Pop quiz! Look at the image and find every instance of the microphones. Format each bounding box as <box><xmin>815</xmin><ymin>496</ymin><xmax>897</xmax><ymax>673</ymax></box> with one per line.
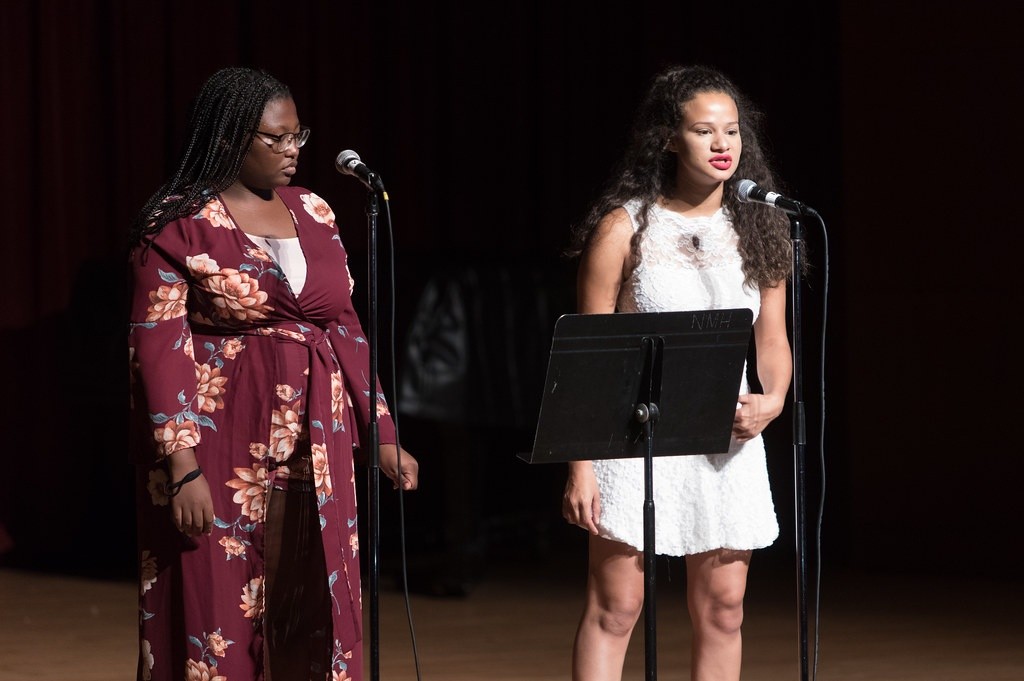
<box><xmin>733</xmin><ymin>178</ymin><xmax>818</xmax><ymax>217</ymax></box>
<box><xmin>335</xmin><ymin>150</ymin><xmax>385</xmax><ymax>193</ymax></box>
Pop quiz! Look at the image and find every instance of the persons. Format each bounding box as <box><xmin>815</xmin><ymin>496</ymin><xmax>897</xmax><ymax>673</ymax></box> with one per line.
<box><xmin>122</xmin><ymin>67</ymin><xmax>419</xmax><ymax>681</ymax></box>
<box><xmin>564</xmin><ymin>66</ymin><xmax>794</xmax><ymax>680</ymax></box>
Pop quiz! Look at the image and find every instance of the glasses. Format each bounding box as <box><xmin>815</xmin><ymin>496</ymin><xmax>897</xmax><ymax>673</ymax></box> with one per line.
<box><xmin>253</xmin><ymin>127</ymin><xmax>310</xmax><ymax>153</ymax></box>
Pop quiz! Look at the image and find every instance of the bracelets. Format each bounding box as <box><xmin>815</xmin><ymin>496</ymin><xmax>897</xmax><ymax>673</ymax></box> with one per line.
<box><xmin>163</xmin><ymin>467</ymin><xmax>201</xmax><ymax>495</ymax></box>
